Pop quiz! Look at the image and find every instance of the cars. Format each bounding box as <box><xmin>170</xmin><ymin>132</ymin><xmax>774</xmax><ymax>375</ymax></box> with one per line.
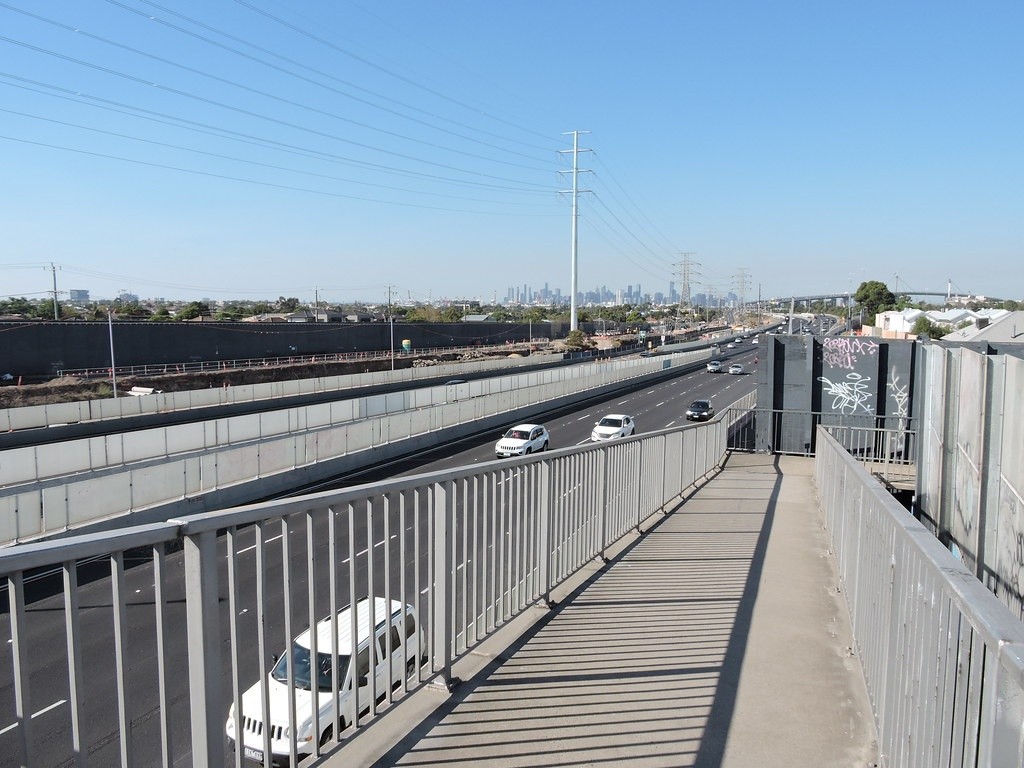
<box><xmin>494</xmin><ymin>424</ymin><xmax>550</xmax><ymax>463</ymax></box>
<box><xmin>686</xmin><ymin>400</ymin><xmax>714</xmax><ymax>422</ymax></box>
<box><xmin>754</xmin><ymin>353</ymin><xmax>759</xmax><ymax>364</ymax></box>
<box><xmin>734</xmin><ymin>338</ymin><xmax>743</xmax><ymax>343</ymax></box>
<box><xmin>744</xmin><ymin>315</ymin><xmax>789</xmax><ymax>345</ymax></box>
<box><xmin>727</xmin><ymin>343</ymin><xmax>737</xmax><ymax>349</ymax></box>
<box><xmin>802</xmin><ymin>316</ymin><xmax>835</xmax><ymax>335</ymax></box>
<box><xmin>729</xmin><ymin>364</ymin><xmax>745</xmax><ymax>375</ymax></box>
<box><xmin>591</xmin><ymin>413</ymin><xmax>635</xmax><ymax>443</ymax></box>
<box><xmin>706</xmin><ymin>361</ymin><xmax>722</xmax><ymax>373</ymax></box>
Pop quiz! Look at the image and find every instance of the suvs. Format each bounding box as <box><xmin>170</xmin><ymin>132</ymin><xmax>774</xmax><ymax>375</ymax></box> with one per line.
<box><xmin>224</xmin><ymin>595</ymin><xmax>426</xmax><ymax>768</ymax></box>
